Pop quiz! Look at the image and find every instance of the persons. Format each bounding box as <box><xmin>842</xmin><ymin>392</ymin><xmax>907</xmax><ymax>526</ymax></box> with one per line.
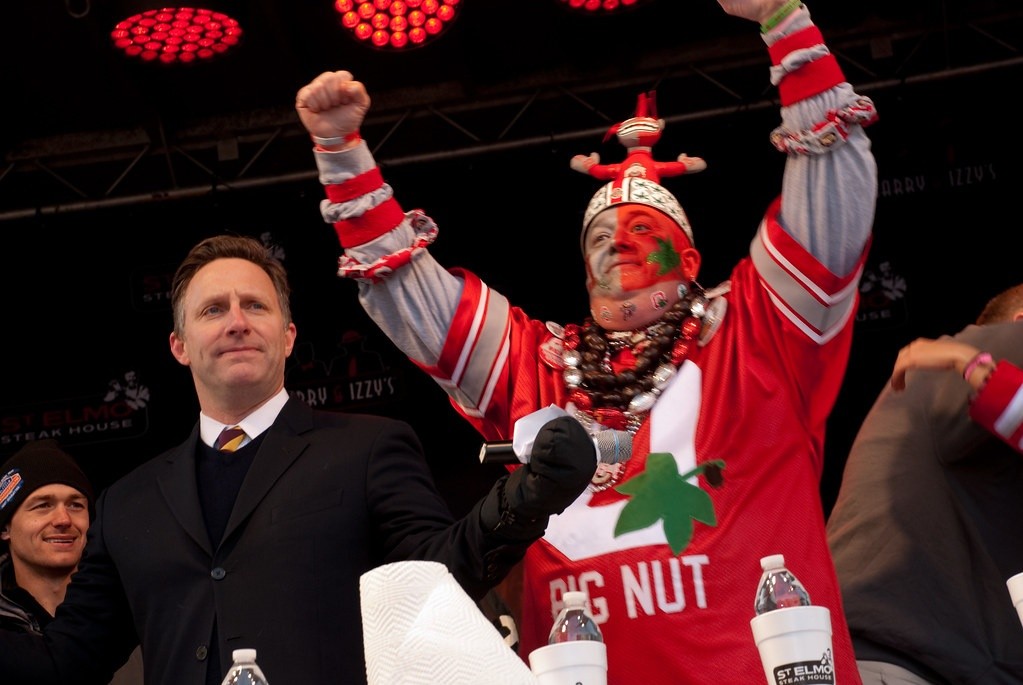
<box><xmin>824</xmin><ymin>284</ymin><xmax>1023</xmax><ymax>683</ymax></box>
<box><xmin>0</xmin><ymin>445</ymin><xmax>89</xmax><ymax>665</ymax></box>
<box><xmin>890</xmin><ymin>336</ymin><xmax>1022</xmax><ymax>452</ymax></box>
<box><xmin>0</xmin><ymin>235</ymin><xmax>595</xmax><ymax>685</ymax></box>
<box><xmin>292</xmin><ymin>0</ymin><xmax>872</xmax><ymax>685</ymax></box>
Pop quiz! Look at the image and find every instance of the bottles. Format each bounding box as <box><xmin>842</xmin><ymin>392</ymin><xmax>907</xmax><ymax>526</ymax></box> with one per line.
<box><xmin>754</xmin><ymin>554</ymin><xmax>811</xmax><ymax>616</ymax></box>
<box><xmin>222</xmin><ymin>648</ymin><xmax>269</xmax><ymax>685</ymax></box>
<box><xmin>549</xmin><ymin>592</ymin><xmax>603</xmax><ymax>643</ymax></box>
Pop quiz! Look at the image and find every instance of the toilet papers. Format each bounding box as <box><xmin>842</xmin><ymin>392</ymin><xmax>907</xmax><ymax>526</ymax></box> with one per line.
<box><xmin>360</xmin><ymin>560</ymin><xmax>539</xmax><ymax>685</ymax></box>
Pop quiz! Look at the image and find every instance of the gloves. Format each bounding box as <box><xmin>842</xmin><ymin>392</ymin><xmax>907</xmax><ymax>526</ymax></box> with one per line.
<box><xmin>481</xmin><ymin>415</ymin><xmax>597</xmax><ymax>550</ymax></box>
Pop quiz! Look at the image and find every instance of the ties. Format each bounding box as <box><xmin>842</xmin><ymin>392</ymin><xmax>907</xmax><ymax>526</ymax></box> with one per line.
<box><xmin>217</xmin><ymin>425</ymin><xmax>246</xmax><ymax>453</ymax></box>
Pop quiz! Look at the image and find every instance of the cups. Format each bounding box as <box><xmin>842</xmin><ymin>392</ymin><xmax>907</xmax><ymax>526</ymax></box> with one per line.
<box><xmin>529</xmin><ymin>640</ymin><xmax>608</xmax><ymax>685</ymax></box>
<box><xmin>750</xmin><ymin>606</ymin><xmax>836</xmax><ymax>685</ymax></box>
<box><xmin>1006</xmin><ymin>572</ymin><xmax>1023</xmax><ymax>628</ymax></box>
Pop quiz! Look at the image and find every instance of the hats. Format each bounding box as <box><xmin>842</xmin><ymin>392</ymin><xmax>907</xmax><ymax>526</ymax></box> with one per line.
<box><xmin>570</xmin><ymin>90</ymin><xmax>708</xmax><ymax>254</ymax></box>
<box><xmin>0</xmin><ymin>438</ymin><xmax>94</xmax><ymax>527</ymax></box>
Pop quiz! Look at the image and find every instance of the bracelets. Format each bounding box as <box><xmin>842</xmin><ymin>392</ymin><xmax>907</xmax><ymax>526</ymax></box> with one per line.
<box><xmin>962</xmin><ymin>350</ymin><xmax>993</xmax><ymax>383</ymax></box>
<box><xmin>760</xmin><ymin>0</ymin><xmax>805</xmax><ymax>33</ymax></box>
<box><xmin>310</xmin><ymin>130</ymin><xmax>360</xmax><ymax>146</ymax></box>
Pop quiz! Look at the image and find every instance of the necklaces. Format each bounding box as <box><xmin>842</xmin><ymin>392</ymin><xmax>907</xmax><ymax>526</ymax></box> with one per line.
<box><xmin>559</xmin><ymin>291</ymin><xmax>707</xmax><ymax>492</ymax></box>
